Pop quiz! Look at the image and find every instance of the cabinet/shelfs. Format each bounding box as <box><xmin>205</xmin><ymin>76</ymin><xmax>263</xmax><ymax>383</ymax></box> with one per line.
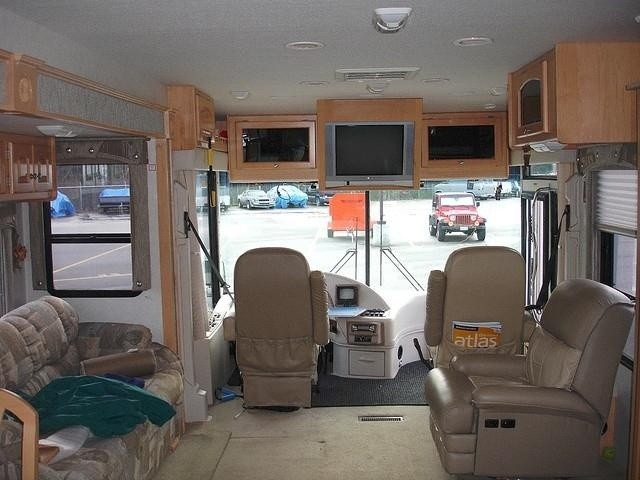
<box><xmin>508</xmin><ymin>41</ymin><xmax>640</xmax><ymax>150</ymax></box>
<box><xmin>168</xmin><ymin>86</ymin><xmax>216</xmax><ymax>151</ymax></box>
<box><xmin>226</xmin><ymin>114</ymin><xmax>319</xmax><ymax>182</ymax></box>
<box><xmin>421</xmin><ymin>112</ymin><xmax>510</xmax><ymax>180</ymax></box>
<box><xmin>0</xmin><ymin>131</ymin><xmax>57</xmax><ymax>203</ymax></box>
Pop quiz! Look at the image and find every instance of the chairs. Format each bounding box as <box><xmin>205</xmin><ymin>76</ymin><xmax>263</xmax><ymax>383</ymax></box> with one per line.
<box><xmin>0</xmin><ymin>387</ymin><xmax>39</xmax><ymax>480</ymax></box>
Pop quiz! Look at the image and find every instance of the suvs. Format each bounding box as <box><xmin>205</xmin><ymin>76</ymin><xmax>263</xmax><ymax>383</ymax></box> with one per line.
<box><xmin>429</xmin><ymin>193</ymin><xmax>487</xmax><ymax>241</ymax></box>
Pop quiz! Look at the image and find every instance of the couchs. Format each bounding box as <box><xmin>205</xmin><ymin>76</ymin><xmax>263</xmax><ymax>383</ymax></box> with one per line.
<box><xmin>424</xmin><ymin>277</ymin><xmax>637</xmax><ymax>480</ymax></box>
<box><xmin>0</xmin><ymin>296</ymin><xmax>187</xmax><ymax>480</ymax></box>
<box><xmin>221</xmin><ymin>247</ymin><xmax>330</xmax><ymax>409</ymax></box>
<box><xmin>425</xmin><ymin>247</ymin><xmax>537</xmax><ymax>369</ymax></box>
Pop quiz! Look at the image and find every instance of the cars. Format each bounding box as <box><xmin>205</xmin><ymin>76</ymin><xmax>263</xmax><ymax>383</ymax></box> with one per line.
<box><xmin>268</xmin><ymin>185</ymin><xmax>309</xmax><ymax>208</ymax></box>
<box><xmin>236</xmin><ymin>190</ymin><xmax>275</xmax><ymax>209</ymax></box>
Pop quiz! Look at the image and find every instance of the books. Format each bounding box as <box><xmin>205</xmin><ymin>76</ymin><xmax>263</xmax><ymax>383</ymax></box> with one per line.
<box><xmin>327</xmin><ymin>307</ymin><xmax>366</xmax><ymax>317</ymax></box>
<box><xmin>452</xmin><ymin>321</ymin><xmax>502</xmax><ymax>348</ymax></box>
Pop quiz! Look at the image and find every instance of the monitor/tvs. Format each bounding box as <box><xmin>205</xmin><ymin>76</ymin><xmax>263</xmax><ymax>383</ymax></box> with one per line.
<box><xmin>323</xmin><ymin>119</ymin><xmax>416</xmax><ymax>188</ymax></box>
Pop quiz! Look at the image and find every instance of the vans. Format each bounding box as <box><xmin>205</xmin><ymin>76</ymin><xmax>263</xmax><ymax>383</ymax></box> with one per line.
<box><xmin>433</xmin><ymin>180</ymin><xmax>502</xmax><ymax>200</ymax></box>
<box><xmin>305</xmin><ymin>190</ymin><xmax>333</xmax><ymax>207</ymax></box>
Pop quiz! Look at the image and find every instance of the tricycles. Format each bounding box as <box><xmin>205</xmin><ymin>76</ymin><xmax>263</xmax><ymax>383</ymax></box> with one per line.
<box><xmin>326</xmin><ymin>194</ymin><xmax>376</xmax><ymax>239</ymax></box>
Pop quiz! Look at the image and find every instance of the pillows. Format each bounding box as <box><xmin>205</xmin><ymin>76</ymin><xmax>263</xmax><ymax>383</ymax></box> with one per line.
<box><xmin>81</xmin><ymin>347</ymin><xmax>156</xmax><ymax>379</ymax></box>
